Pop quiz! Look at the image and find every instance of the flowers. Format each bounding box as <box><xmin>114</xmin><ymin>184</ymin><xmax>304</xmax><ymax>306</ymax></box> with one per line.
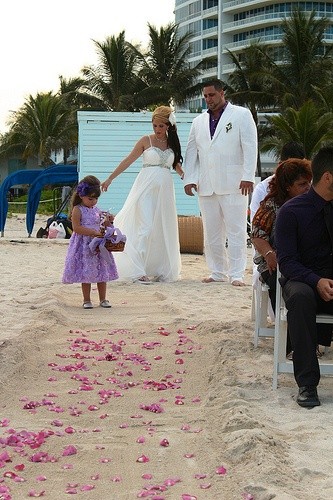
<box><xmin>169</xmin><ymin>112</ymin><xmax>177</xmax><ymax>125</ymax></box>
<box><xmin>76</xmin><ymin>182</ymin><xmax>87</xmax><ymax>194</ymax></box>
<box><xmin>224</xmin><ymin>123</ymin><xmax>233</xmax><ymax>133</ymax></box>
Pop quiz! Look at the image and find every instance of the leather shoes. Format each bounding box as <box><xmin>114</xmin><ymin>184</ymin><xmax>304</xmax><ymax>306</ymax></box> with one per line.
<box><xmin>297</xmin><ymin>386</ymin><xmax>320</xmax><ymax>406</ymax></box>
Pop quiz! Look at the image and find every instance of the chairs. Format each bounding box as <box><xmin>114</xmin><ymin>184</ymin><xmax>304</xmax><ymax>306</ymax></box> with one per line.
<box><xmin>249</xmin><ymin>173</ymin><xmax>333</xmax><ymax>389</ymax></box>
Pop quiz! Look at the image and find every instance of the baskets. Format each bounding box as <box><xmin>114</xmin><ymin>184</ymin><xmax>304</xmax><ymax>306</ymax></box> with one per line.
<box><xmin>95</xmin><ymin>225</ymin><xmax>126</xmax><ymax>251</ymax></box>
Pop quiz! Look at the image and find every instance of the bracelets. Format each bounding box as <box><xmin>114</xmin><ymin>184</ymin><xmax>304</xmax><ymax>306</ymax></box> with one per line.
<box><xmin>265</xmin><ymin>250</ymin><xmax>275</xmax><ymax>259</ymax></box>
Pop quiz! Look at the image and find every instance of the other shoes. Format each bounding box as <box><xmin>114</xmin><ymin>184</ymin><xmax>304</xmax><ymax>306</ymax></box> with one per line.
<box><xmin>100</xmin><ymin>300</ymin><xmax>111</xmax><ymax>307</ymax></box>
<box><xmin>83</xmin><ymin>302</ymin><xmax>92</xmax><ymax>309</ymax></box>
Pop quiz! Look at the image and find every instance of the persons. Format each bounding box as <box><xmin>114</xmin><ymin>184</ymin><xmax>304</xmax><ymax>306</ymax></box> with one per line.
<box><xmin>272</xmin><ymin>147</ymin><xmax>333</xmax><ymax>408</ymax></box>
<box><xmin>101</xmin><ymin>106</ymin><xmax>184</xmax><ymax>284</ymax></box>
<box><xmin>183</xmin><ymin>78</ymin><xmax>258</xmax><ymax>286</ymax></box>
<box><xmin>61</xmin><ymin>175</ymin><xmax>120</xmax><ymax>309</ymax></box>
<box><xmin>250</xmin><ymin>158</ymin><xmax>313</xmax><ymax>360</ymax></box>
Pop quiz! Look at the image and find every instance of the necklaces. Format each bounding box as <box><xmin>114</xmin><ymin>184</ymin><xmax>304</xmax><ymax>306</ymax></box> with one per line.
<box><xmin>154</xmin><ymin>134</ymin><xmax>168</xmax><ymax>142</ymax></box>
